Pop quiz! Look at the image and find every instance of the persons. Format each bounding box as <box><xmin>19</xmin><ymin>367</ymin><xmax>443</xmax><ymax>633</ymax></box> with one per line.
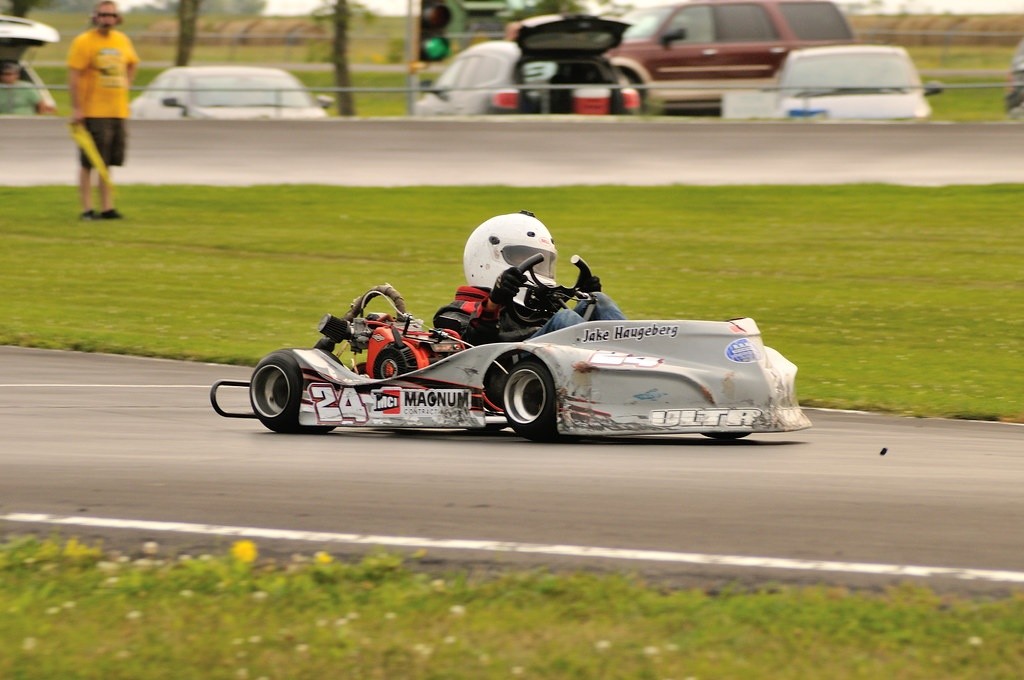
<box><xmin>1008</xmin><ymin>37</ymin><xmax>1024</xmax><ymax>120</ymax></box>
<box><xmin>0</xmin><ymin>63</ymin><xmax>41</xmax><ymax>116</ymax></box>
<box><xmin>65</xmin><ymin>0</ymin><xmax>138</xmax><ymax>220</ymax></box>
<box><xmin>432</xmin><ymin>210</ymin><xmax>627</xmax><ymax>349</ymax></box>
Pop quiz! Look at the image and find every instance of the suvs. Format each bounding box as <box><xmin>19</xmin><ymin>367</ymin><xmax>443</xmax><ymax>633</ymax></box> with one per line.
<box><xmin>416</xmin><ymin>12</ymin><xmax>643</xmax><ymax>115</ymax></box>
<box><xmin>603</xmin><ymin>1</ymin><xmax>856</xmax><ymax>104</ymax></box>
<box><xmin>0</xmin><ymin>16</ymin><xmax>60</xmax><ymax>117</ymax></box>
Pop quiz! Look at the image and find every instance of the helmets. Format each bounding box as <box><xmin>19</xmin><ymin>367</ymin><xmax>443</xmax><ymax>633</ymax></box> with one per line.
<box><xmin>464</xmin><ymin>212</ymin><xmax>557</xmax><ymax>306</ymax></box>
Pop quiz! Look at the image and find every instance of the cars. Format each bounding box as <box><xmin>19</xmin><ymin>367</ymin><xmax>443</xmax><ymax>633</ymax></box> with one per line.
<box><xmin>762</xmin><ymin>46</ymin><xmax>944</xmax><ymax>121</ymax></box>
<box><xmin>128</xmin><ymin>65</ymin><xmax>335</xmax><ymax>121</ymax></box>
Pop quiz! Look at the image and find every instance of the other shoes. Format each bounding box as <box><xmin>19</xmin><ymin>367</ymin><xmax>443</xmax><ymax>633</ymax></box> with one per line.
<box><xmin>82</xmin><ymin>210</ymin><xmax>94</xmax><ymax>219</ymax></box>
<box><xmin>99</xmin><ymin>208</ymin><xmax>122</xmax><ymax>218</ymax></box>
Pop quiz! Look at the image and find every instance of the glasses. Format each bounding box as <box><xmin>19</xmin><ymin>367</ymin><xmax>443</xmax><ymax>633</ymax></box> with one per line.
<box><xmin>98</xmin><ymin>12</ymin><xmax>118</xmax><ymax>18</ymax></box>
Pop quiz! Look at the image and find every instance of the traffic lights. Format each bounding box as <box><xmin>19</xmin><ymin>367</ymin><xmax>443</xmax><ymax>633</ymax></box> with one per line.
<box><xmin>411</xmin><ymin>1</ymin><xmax>453</xmax><ymax>64</ymax></box>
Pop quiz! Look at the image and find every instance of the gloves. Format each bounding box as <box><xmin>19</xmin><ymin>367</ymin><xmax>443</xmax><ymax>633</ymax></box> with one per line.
<box><xmin>576</xmin><ymin>276</ymin><xmax>602</xmax><ymax>294</ymax></box>
<box><xmin>489</xmin><ymin>266</ymin><xmax>528</xmax><ymax>307</ymax></box>
